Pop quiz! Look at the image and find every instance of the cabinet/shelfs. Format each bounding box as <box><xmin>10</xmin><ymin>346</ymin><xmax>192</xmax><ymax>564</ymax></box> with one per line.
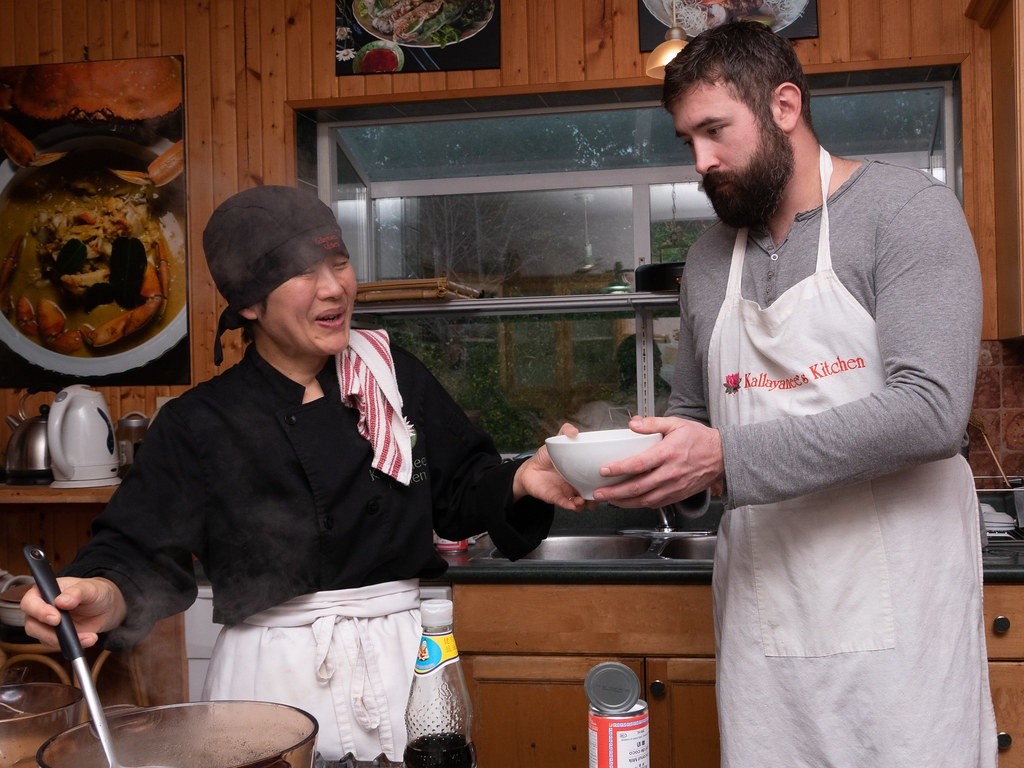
<box><xmin>452</xmin><ymin>584</ymin><xmax>1024</xmax><ymax>768</ymax></box>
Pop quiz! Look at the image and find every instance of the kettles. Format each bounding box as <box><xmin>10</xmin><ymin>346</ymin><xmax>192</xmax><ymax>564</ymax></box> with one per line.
<box><xmin>1</xmin><ymin>385</ymin><xmax>65</xmax><ymax>485</ymax></box>
<box><xmin>46</xmin><ymin>384</ymin><xmax>123</xmax><ymax>487</ymax></box>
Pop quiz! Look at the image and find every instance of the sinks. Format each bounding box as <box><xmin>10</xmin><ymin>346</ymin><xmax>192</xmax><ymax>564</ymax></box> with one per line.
<box><xmin>466</xmin><ymin>530</ymin><xmax>658</xmax><ymax>563</ymax></box>
<box><xmin>658</xmin><ymin>531</ymin><xmax>719</xmax><ymax>563</ymax></box>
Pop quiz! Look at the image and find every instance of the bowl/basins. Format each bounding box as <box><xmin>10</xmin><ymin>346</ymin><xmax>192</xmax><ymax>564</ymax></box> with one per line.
<box><xmin>35</xmin><ymin>700</ymin><xmax>319</xmax><ymax>768</ymax></box>
<box><xmin>0</xmin><ymin>681</ymin><xmax>86</xmax><ymax>768</ymax></box>
<box><xmin>545</xmin><ymin>427</ymin><xmax>666</xmax><ymax>501</ymax></box>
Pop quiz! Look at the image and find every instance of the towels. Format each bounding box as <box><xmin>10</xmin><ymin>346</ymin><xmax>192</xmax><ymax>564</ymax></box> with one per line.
<box><xmin>331</xmin><ymin>322</ymin><xmax>417</xmax><ymax>486</ymax></box>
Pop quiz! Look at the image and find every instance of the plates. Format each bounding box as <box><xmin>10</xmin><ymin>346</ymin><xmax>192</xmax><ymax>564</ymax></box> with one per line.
<box><xmin>980</xmin><ymin>503</ymin><xmax>1017</xmax><ymax>532</ymax></box>
<box><xmin>0</xmin><ymin>137</ymin><xmax>187</xmax><ymax>380</ymax></box>
<box><xmin>640</xmin><ymin>0</ymin><xmax>810</xmax><ymax>42</ymax></box>
<box><xmin>351</xmin><ymin>0</ymin><xmax>496</xmax><ymax>47</ymax></box>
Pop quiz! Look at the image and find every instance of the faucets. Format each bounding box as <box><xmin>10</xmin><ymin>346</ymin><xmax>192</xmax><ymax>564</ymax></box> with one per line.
<box><xmin>657</xmin><ymin>506</ymin><xmax>675</xmax><ymax>532</ymax></box>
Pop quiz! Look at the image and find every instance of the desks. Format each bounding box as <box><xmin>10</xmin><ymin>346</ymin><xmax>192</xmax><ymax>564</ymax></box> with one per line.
<box><xmin>0</xmin><ymin>484</ymin><xmax>189</xmax><ymax>707</ymax></box>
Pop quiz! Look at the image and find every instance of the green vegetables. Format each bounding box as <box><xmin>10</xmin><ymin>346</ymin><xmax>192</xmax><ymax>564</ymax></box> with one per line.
<box><xmin>404</xmin><ymin>0</ymin><xmax>490</xmax><ymax>48</ymax></box>
<box><xmin>395</xmin><ymin>325</ymin><xmax>548</xmax><ymax>452</ymax></box>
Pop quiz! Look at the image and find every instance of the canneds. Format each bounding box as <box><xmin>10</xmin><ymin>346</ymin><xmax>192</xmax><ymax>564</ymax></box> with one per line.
<box><xmin>582</xmin><ymin>660</ymin><xmax>650</xmax><ymax>768</ymax></box>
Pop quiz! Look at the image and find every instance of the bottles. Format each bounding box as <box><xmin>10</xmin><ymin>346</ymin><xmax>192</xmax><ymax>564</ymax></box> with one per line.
<box><xmin>403</xmin><ymin>597</ymin><xmax>479</xmax><ymax>767</ymax></box>
<box><xmin>116</xmin><ymin>418</ymin><xmax>149</xmax><ymax>477</ymax></box>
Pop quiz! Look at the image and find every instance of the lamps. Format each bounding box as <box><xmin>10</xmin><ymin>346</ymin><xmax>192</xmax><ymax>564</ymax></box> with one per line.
<box><xmin>604</xmin><ymin>261</ymin><xmax>634</xmax><ymax>294</ymax></box>
<box><xmin>646</xmin><ymin>0</ymin><xmax>689</xmax><ymax>79</ymax></box>
<box><xmin>574</xmin><ymin>190</ymin><xmax>600</xmax><ymax>273</ymax></box>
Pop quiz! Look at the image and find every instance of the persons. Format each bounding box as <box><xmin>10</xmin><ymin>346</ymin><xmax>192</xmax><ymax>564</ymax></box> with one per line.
<box><xmin>586</xmin><ymin>20</ymin><xmax>996</xmax><ymax>768</ymax></box>
<box><xmin>19</xmin><ymin>184</ymin><xmax>592</xmax><ymax>766</ymax></box>
<box><xmin>609</xmin><ymin>332</ymin><xmax>676</xmax><ymax>421</ymax></box>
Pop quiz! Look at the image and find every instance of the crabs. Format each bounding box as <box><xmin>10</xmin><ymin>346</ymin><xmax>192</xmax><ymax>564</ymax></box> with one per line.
<box><xmin>0</xmin><ymin>57</ymin><xmax>185</xmax><ymax>188</ymax></box>
<box><xmin>0</xmin><ymin>233</ymin><xmax>170</xmax><ymax>351</ymax></box>
